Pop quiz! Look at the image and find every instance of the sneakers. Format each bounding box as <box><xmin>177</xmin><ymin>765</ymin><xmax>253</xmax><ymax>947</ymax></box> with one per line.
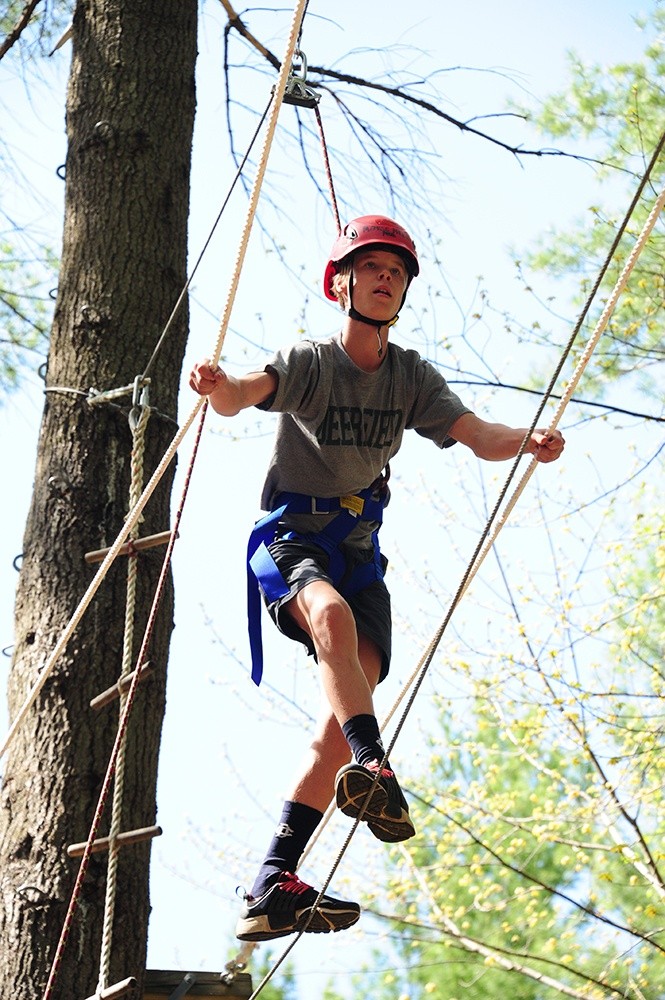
<box><xmin>236</xmin><ymin>872</ymin><xmax>361</xmax><ymax>941</ymax></box>
<box><xmin>334</xmin><ymin>763</ymin><xmax>416</xmax><ymax>842</ymax></box>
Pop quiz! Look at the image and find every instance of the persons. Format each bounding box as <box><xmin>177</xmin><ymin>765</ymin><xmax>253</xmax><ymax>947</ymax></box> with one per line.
<box><xmin>189</xmin><ymin>215</ymin><xmax>566</xmax><ymax>942</ymax></box>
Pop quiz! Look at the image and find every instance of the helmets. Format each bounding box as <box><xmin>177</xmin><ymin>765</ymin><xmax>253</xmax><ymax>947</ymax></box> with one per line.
<box><xmin>324</xmin><ymin>215</ymin><xmax>419</xmax><ymax>300</ymax></box>
